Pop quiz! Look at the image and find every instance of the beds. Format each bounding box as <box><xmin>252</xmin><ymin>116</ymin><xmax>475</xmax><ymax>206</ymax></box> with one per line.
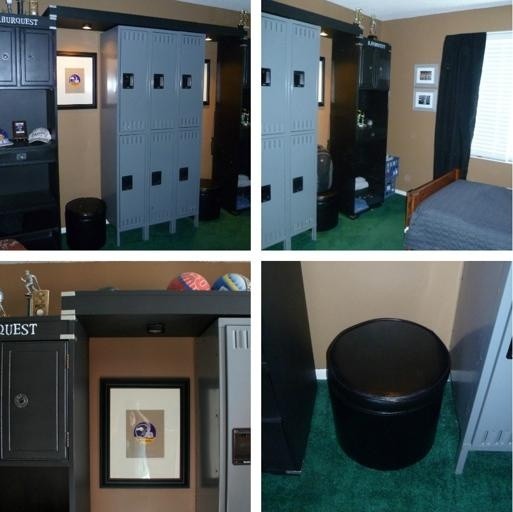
<box><xmin>399</xmin><ymin>167</ymin><xmax>512</xmax><ymax>252</ymax></box>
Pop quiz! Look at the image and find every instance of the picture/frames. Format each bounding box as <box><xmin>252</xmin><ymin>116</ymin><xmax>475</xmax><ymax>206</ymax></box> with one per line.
<box><xmin>317</xmin><ymin>56</ymin><xmax>325</xmax><ymax>108</ymax></box>
<box><xmin>54</xmin><ymin>49</ymin><xmax>98</xmax><ymax>111</ymax></box>
<box><xmin>412</xmin><ymin>62</ymin><xmax>439</xmax><ymax>89</ymax></box>
<box><xmin>96</xmin><ymin>372</ymin><xmax>191</xmax><ymax>489</ymax></box>
<box><xmin>411</xmin><ymin>87</ymin><xmax>438</xmax><ymax>114</ymax></box>
<box><xmin>200</xmin><ymin>57</ymin><xmax>211</xmax><ymax>107</ymax></box>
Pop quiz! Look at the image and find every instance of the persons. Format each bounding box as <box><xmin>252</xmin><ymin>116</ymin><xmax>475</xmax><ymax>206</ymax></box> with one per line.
<box><xmin>21</xmin><ymin>269</ymin><xmax>37</xmax><ymax>295</ymax></box>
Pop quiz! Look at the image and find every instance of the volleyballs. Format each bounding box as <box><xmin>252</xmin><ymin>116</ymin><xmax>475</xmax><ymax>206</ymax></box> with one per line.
<box><xmin>134</xmin><ymin>422</ymin><xmax>157</xmax><ymax>443</ymax></box>
<box><xmin>69</xmin><ymin>74</ymin><xmax>80</xmax><ymax>88</ymax></box>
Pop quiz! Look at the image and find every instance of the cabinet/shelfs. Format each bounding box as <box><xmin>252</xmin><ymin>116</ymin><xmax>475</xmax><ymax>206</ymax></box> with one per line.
<box><xmin>0</xmin><ymin>4</ymin><xmax>63</xmax><ymax>252</ymax></box>
<box><xmin>94</xmin><ymin>23</ymin><xmax>207</xmax><ymax>247</ymax></box>
<box><xmin>190</xmin><ymin>316</ymin><xmax>251</xmax><ymax>512</ymax></box>
<box><xmin>0</xmin><ymin>313</ymin><xmax>91</xmax><ymax>511</ymax></box>
<box><xmin>327</xmin><ymin>34</ymin><xmax>392</xmax><ymax>220</ymax></box>
<box><xmin>447</xmin><ymin>260</ymin><xmax>512</xmax><ymax>477</ymax></box>
<box><xmin>257</xmin><ymin>11</ymin><xmax>324</xmax><ymax>251</ymax></box>
<box><xmin>259</xmin><ymin>260</ymin><xmax>318</xmax><ymax>477</ymax></box>
<box><xmin>210</xmin><ymin>36</ymin><xmax>250</xmax><ymax>217</ymax></box>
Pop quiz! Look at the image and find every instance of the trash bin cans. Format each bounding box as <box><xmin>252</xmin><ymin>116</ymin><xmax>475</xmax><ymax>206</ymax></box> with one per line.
<box><xmin>308</xmin><ymin>189</ymin><xmax>339</xmax><ymax>232</ymax></box>
<box><xmin>189</xmin><ymin>178</ymin><xmax>220</xmax><ymax>221</ymax></box>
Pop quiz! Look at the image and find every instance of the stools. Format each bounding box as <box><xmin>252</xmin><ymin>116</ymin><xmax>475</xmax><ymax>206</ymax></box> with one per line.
<box><xmin>315</xmin><ymin>186</ymin><xmax>339</xmax><ymax>234</ymax></box>
<box><xmin>186</xmin><ymin>176</ymin><xmax>223</xmax><ymax>222</ymax></box>
